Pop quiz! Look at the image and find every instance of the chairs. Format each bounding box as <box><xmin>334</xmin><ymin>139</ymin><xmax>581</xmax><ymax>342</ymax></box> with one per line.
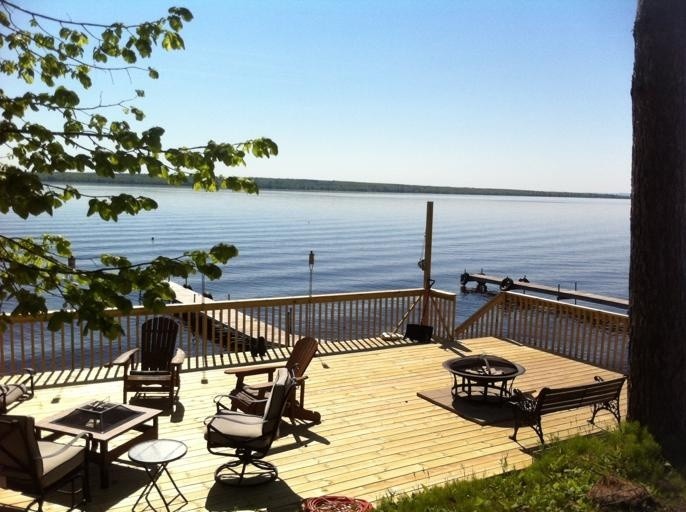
<box><xmin>0</xmin><ymin>413</ymin><xmax>97</xmax><ymax>512</ymax></box>
<box><xmin>0</xmin><ymin>366</ymin><xmax>36</xmax><ymax>415</ymax></box>
<box><xmin>112</xmin><ymin>317</ymin><xmax>186</xmax><ymax>404</ymax></box>
<box><xmin>203</xmin><ymin>336</ymin><xmax>322</xmax><ymax>489</ymax></box>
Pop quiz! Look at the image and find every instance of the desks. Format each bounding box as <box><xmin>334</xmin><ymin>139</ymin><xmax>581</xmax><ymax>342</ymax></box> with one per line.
<box><xmin>35</xmin><ymin>399</ymin><xmax>166</xmax><ymax>489</ymax></box>
<box><xmin>443</xmin><ymin>354</ymin><xmax>526</xmax><ymax>407</ymax></box>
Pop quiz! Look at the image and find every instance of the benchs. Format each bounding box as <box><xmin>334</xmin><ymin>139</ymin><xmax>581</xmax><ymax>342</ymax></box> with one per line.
<box><xmin>508</xmin><ymin>373</ymin><xmax>626</xmax><ymax>452</ymax></box>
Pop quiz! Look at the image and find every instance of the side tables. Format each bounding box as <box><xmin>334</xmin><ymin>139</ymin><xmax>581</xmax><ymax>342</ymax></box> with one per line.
<box><xmin>128</xmin><ymin>438</ymin><xmax>191</xmax><ymax>512</ymax></box>
